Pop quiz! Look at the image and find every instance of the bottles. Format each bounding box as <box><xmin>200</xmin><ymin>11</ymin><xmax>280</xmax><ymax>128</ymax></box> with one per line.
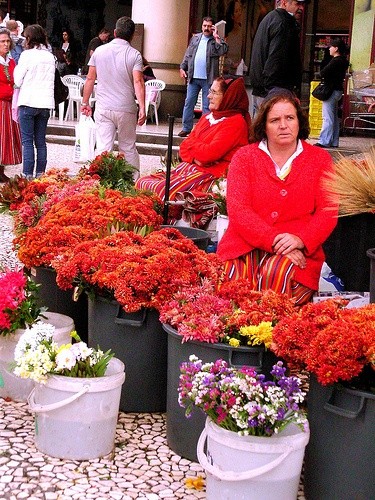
<box><xmin>314</xmin><ymin>34</ymin><xmax>349</xmax><ymax>81</ymax></box>
<box><xmin>77</xmin><ymin>68</ymin><xmax>82</xmax><ymax>77</ymax></box>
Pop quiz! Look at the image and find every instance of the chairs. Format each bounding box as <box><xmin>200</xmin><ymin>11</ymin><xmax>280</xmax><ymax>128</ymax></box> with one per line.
<box><xmin>135</xmin><ymin>79</ymin><xmax>166</xmax><ymax>127</ymax></box>
<box><xmin>60</xmin><ymin>75</ymin><xmax>86</xmax><ymax>121</ymax></box>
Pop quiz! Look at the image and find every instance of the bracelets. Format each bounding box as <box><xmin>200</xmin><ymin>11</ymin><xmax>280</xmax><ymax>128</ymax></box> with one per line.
<box><xmin>81</xmin><ymin>103</ymin><xmax>89</xmax><ymax>106</ymax></box>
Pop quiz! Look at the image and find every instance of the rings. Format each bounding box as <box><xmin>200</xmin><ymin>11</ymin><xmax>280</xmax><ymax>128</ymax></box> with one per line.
<box><xmin>282</xmin><ymin>245</ymin><xmax>286</xmax><ymax>249</ymax></box>
<box><xmin>290</xmin><ymin>253</ymin><xmax>295</xmax><ymax>255</ymax></box>
<box><xmin>294</xmin><ymin>251</ymin><xmax>298</xmax><ymax>254</ymax></box>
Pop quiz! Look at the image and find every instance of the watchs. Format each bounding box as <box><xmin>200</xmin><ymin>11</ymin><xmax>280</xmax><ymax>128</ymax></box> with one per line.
<box><xmin>215</xmin><ymin>35</ymin><xmax>221</xmax><ymax>39</ymax></box>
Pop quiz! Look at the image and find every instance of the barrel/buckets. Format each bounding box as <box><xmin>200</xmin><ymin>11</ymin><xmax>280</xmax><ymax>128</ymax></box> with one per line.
<box><xmin>30</xmin><ymin>266</ymin><xmax>87</xmax><ymax>345</ymax></box>
<box><xmin>159</xmin><ymin>225</ymin><xmax>210</xmax><ymax>254</ymax></box>
<box><xmin>196</xmin><ymin>415</ymin><xmax>310</xmax><ymax>500</ymax></box>
<box><xmin>162</xmin><ymin>323</ymin><xmax>287</xmax><ymax>463</ymax></box>
<box><xmin>0</xmin><ymin>311</ymin><xmax>75</xmax><ymax>402</ymax></box>
<box><xmin>84</xmin><ymin>289</ymin><xmax>166</xmax><ymax>413</ymax></box>
<box><xmin>304</xmin><ymin>370</ymin><xmax>375</xmax><ymax>500</ymax></box>
<box><xmin>27</xmin><ymin>352</ymin><xmax>125</xmax><ymax>459</ymax></box>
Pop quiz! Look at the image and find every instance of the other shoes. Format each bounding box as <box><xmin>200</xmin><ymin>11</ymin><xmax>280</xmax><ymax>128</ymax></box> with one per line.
<box><xmin>178</xmin><ymin>130</ymin><xmax>191</xmax><ymax>137</ymax></box>
<box><xmin>0</xmin><ymin>165</ymin><xmax>10</xmax><ymax>183</ymax></box>
<box><xmin>329</xmin><ymin>145</ymin><xmax>338</xmax><ymax>149</ymax></box>
<box><xmin>312</xmin><ymin>143</ymin><xmax>328</xmax><ymax>148</ymax></box>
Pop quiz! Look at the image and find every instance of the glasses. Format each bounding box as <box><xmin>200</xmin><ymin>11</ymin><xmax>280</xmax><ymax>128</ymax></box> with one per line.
<box><xmin>208</xmin><ymin>88</ymin><xmax>223</xmax><ymax>95</ymax></box>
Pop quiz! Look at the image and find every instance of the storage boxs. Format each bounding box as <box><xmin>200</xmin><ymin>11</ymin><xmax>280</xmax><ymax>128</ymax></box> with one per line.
<box><xmin>309</xmin><ymin>81</ymin><xmax>322</xmax><ymax>138</ymax></box>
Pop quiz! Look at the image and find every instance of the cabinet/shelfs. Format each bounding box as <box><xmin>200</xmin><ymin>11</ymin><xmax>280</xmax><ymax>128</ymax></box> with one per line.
<box><xmin>312</xmin><ymin>34</ymin><xmax>351</xmax><ymax>83</ymax></box>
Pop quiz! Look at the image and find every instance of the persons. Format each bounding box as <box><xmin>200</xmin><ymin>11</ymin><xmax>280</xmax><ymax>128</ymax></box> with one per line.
<box><xmin>0</xmin><ymin>7</ymin><xmax>86</xmax><ymax>120</ymax></box>
<box><xmin>313</xmin><ymin>40</ymin><xmax>350</xmax><ymax>148</ymax></box>
<box><xmin>13</xmin><ymin>24</ymin><xmax>57</xmax><ymax>180</ymax></box>
<box><xmin>0</xmin><ymin>29</ymin><xmax>22</xmax><ymax>183</ymax></box>
<box><xmin>178</xmin><ymin>16</ymin><xmax>230</xmax><ymax>137</ymax></box>
<box><xmin>216</xmin><ymin>87</ymin><xmax>340</xmax><ymax>307</ymax></box>
<box><xmin>134</xmin><ymin>74</ymin><xmax>255</xmax><ymax>229</ymax></box>
<box><xmin>142</xmin><ymin>55</ymin><xmax>156</xmax><ymax>86</ymax></box>
<box><xmin>81</xmin><ymin>16</ymin><xmax>147</xmax><ymax>184</ymax></box>
<box><xmin>250</xmin><ymin>0</ymin><xmax>304</xmax><ymax>119</ymax></box>
<box><xmin>81</xmin><ymin>28</ymin><xmax>113</xmax><ymax>75</ymax></box>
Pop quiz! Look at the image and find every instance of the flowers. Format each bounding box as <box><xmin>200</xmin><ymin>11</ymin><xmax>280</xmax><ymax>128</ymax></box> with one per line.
<box><xmin>1</xmin><ymin>150</ymin><xmax>375</xmax><ymax>437</ymax></box>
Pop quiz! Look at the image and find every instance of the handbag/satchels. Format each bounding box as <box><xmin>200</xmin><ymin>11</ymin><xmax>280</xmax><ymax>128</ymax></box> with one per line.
<box><xmin>312</xmin><ymin>77</ymin><xmax>334</xmax><ymax>101</ymax></box>
